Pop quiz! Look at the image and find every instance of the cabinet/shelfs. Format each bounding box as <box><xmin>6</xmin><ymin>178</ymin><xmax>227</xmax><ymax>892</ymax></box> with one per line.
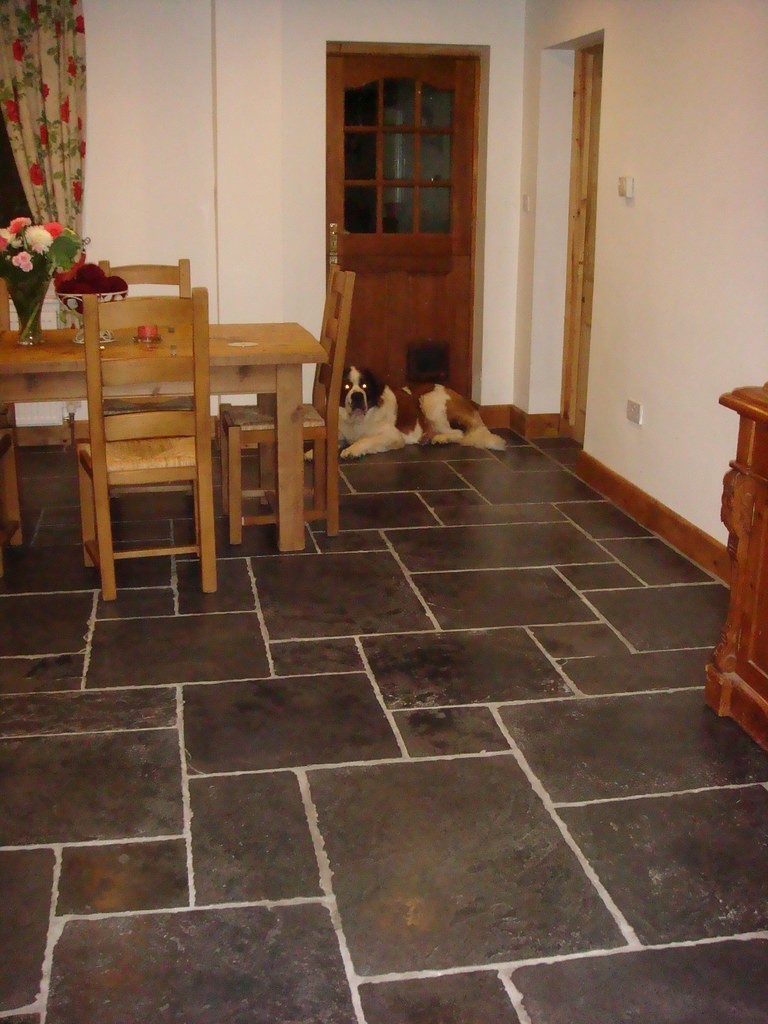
<box><xmin>703</xmin><ymin>382</ymin><xmax>768</xmax><ymax>753</ymax></box>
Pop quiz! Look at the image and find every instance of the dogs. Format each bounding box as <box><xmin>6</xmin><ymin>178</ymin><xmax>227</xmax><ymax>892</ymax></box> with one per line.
<box><xmin>304</xmin><ymin>366</ymin><xmax>506</xmax><ymax>461</ymax></box>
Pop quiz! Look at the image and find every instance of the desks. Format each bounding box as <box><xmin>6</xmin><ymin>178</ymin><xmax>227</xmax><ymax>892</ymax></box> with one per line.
<box><xmin>0</xmin><ymin>323</ymin><xmax>329</xmax><ymax>552</ymax></box>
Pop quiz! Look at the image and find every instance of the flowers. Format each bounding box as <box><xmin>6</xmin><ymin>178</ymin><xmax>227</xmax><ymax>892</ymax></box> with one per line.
<box><xmin>0</xmin><ymin>217</ymin><xmax>85</xmax><ymax>344</ymax></box>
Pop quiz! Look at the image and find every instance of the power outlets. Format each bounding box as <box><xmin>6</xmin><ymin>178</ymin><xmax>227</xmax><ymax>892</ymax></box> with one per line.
<box><xmin>625</xmin><ymin>400</ymin><xmax>643</xmax><ymax>425</ymax></box>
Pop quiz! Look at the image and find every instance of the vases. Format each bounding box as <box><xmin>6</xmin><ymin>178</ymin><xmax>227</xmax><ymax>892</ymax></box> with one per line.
<box><xmin>6</xmin><ymin>279</ymin><xmax>48</xmax><ymax>346</ymax></box>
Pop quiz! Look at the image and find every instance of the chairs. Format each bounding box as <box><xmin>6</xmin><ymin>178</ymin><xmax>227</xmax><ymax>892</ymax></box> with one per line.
<box><xmin>76</xmin><ymin>286</ymin><xmax>217</xmax><ymax>602</ymax></box>
<box><xmin>99</xmin><ymin>259</ymin><xmax>195</xmax><ymax>415</ymax></box>
<box><xmin>219</xmin><ymin>264</ymin><xmax>355</xmax><ymax>545</ymax></box>
